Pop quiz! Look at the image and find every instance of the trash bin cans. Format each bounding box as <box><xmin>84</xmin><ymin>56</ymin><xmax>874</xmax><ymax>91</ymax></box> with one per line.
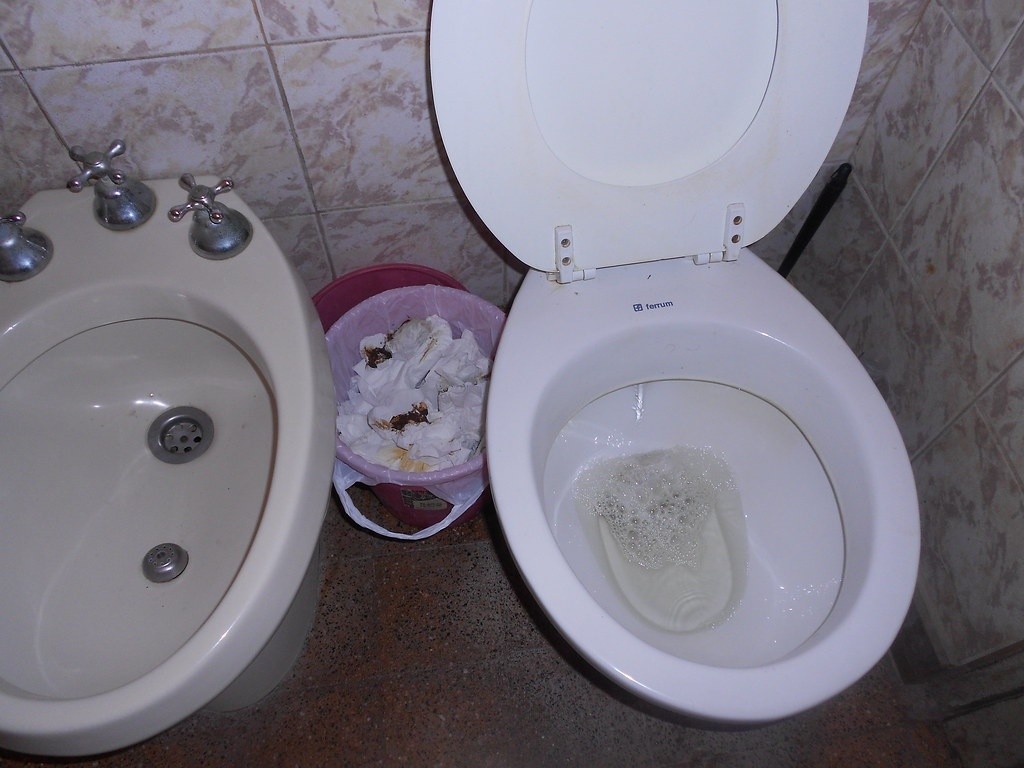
<box><xmin>324</xmin><ymin>284</ymin><xmax>507</xmax><ymax>529</ymax></box>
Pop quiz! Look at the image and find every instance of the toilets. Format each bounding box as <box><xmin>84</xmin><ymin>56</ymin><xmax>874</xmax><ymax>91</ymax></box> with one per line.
<box><xmin>424</xmin><ymin>0</ymin><xmax>923</xmax><ymax>726</ymax></box>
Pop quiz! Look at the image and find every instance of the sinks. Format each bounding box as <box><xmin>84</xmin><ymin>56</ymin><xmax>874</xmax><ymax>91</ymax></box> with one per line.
<box><xmin>3</xmin><ymin>173</ymin><xmax>339</xmax><ymax>762</ymax></box>
